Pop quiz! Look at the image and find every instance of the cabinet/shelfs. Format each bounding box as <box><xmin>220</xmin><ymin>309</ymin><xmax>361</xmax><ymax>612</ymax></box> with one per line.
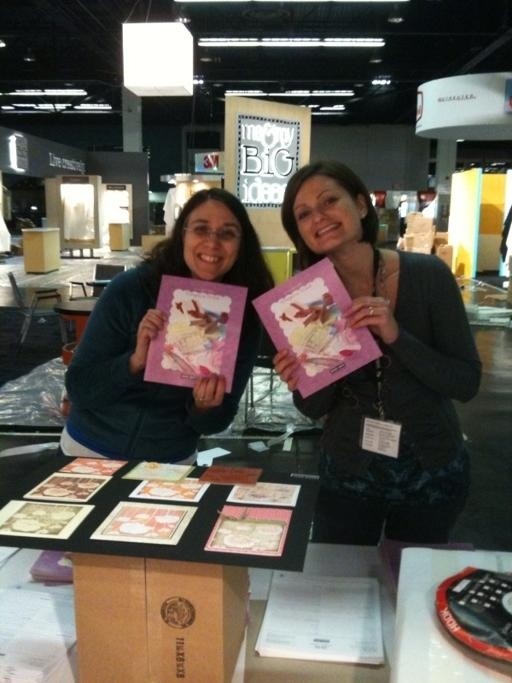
<box><xmin>101</xmin><ymin>183</ymin><xmax>133</xmax><ymax>244</ymax></box>
<box><xmin>45</xmin><ymin>174</ymin><xmax>101</xmax><ymax>258</ymax></box>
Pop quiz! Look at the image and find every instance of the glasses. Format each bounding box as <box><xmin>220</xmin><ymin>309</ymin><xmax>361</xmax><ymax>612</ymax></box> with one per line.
<box><xmin>181</xmin><ymin>218</ymin><xmax>242</xmax><ymax>241</ymax></box>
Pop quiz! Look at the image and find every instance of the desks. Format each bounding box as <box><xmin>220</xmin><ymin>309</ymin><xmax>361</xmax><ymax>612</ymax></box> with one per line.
<box><xmin>54</xmin><ymin>298</ymin><xmax>98</xmax><ymax>365</ymax></box>
<box><xmin>109</xmin><ymin>224</ymin><xmax>131</xmax><ymax>251</ymax></box>
<box><xmin>21</xmin><ymin>228</ymin><xmax>60</xmax><ymax>273</ymax></box>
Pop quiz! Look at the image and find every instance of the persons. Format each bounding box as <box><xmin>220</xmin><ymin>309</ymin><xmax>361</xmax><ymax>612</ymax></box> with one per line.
<box><xmin>51</xmin><ymin>187</ymin><xmax>274</xmax><ymax>469</ymax></box>
<box><xmin>273</xmin><ymin>157</ymin><xmax>481</xmax><ymax>543</ymax></box>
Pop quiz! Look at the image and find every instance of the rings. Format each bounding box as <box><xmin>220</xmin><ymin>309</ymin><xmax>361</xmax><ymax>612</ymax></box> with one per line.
<box><xmin>199</xmin><ymin>397</ymin><xmax>205</xmax><ymax>404</ymax></box>
<box><xmin>367</xmin><ymin>307</ymin><xmax>375</xmax><ymax>317</ymax></box>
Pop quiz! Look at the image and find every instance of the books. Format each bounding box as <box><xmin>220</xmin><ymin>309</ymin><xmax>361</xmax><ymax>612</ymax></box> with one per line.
<box><xmin>142</xmin><ymin>273</ymin><xmax>248</xmax><ymax>395</ymax></box>
<box><xmin>250</xmin><ymin>255</ymin><xmax>383</xmax><ymax>400</ymax></box>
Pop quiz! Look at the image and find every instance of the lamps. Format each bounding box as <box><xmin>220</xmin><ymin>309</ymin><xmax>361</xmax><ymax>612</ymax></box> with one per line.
<box><xmin>122</xmin><ymin>0</ymin><xmax>194</xmax><ymax>97</ymax></box>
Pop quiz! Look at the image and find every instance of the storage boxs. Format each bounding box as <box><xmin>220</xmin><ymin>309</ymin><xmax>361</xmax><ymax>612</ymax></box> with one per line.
<box><xmin>73</xmin><ymin>552</ymin><xmax>249</xmax><ymax>683</ymax></box>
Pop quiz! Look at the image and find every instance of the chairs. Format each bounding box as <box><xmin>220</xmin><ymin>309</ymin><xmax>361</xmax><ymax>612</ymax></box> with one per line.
<box><xmin>69</xmin><ymin>263</ymin><xmax>126</xmax><ymax>298</ymax></box>
<box><xmin>8</xmin><ymin>272</ymin><xmax>68</xmax><ymax>358</ymax></box>
<box><xmin>245</xmin><ymin>326</ymin><xmax>317</xmax><ymax>424</ymax></box>
